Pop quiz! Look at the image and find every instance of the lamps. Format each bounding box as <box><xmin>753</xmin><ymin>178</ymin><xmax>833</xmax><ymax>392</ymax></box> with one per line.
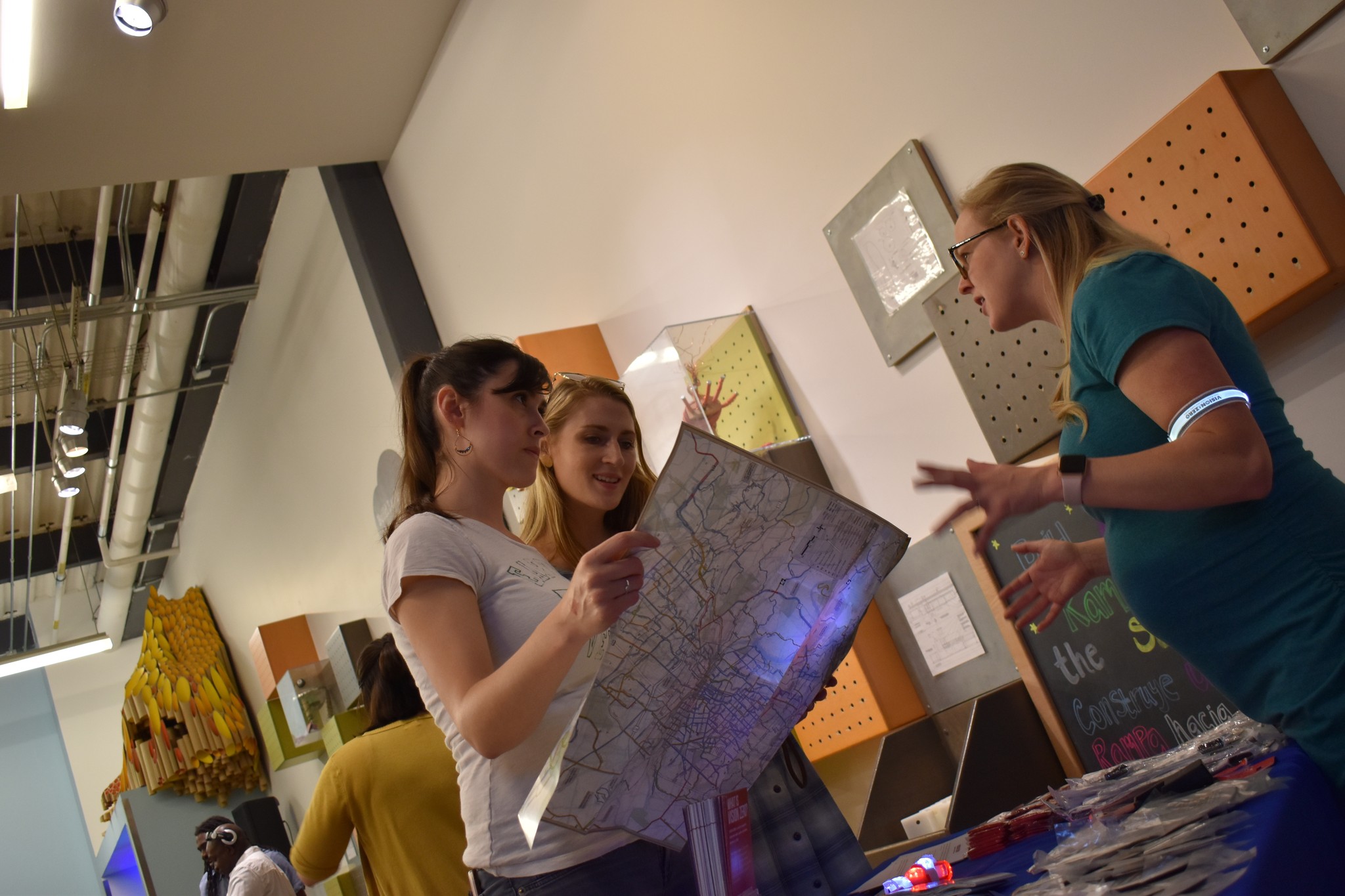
<box><xmin>49</xmin><ymin>380</ymin><xmax>89</xmax><ymax>499</ymax></box>
<box><xmin>114</xmin><ymin>1</ymin><xmax>168</xmax><ymax>36</ymax></box>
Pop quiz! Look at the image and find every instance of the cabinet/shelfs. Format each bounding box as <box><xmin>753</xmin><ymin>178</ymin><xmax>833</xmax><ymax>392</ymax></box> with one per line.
<box><xmin>97</xmin><ymin>782</ymin><xmax>270</xmax><ymax>896</ymax></box>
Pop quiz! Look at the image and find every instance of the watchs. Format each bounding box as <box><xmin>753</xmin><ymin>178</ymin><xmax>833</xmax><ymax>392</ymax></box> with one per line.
<box><xmin>1058</xmin><ymin>454</ymin><xmax>1087</xmax><ymax>507</ymax></box>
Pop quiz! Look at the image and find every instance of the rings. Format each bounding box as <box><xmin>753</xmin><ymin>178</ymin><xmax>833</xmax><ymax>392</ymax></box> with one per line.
<box><xmin>625</xmin><ymin>580</ymin><xmax>629</xmax><ymax>592</ymax></box>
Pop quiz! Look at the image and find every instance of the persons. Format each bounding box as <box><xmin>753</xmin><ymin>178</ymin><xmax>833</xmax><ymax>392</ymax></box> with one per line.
<box><xmin>291</xmin><ymin>634</ymin><xmax>473</xmax><ymax>896</ymax></box>
<box><xmin>520</xmin><ymin>370</ymin><xmax>874</xmax><ymax>895</ymax></box>
<box><xmin>913</xmin><ymin>163</ymin><xmax>1344</xmax><ymax>775</ymax></box>
<box><xmin>194</xmin><ymin>816</ymin><xmax>306</xmax><ymax>896</ymax></box>
<box><xmin>380</xmin><ymin>338</ymin><xmax>691</xmax><ymax>896</ymax></box>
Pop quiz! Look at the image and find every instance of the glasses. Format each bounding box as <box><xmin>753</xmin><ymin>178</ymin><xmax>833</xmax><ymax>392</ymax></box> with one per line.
<box><xmin>946</xmin><ymin>220</ymin><xmax>1009</xmax><ymax>281</ymax></box>
<box><xmin>544</xmin><ymin>371</ymin><xmax>627</xmax><ymax>406</ymax></box>
